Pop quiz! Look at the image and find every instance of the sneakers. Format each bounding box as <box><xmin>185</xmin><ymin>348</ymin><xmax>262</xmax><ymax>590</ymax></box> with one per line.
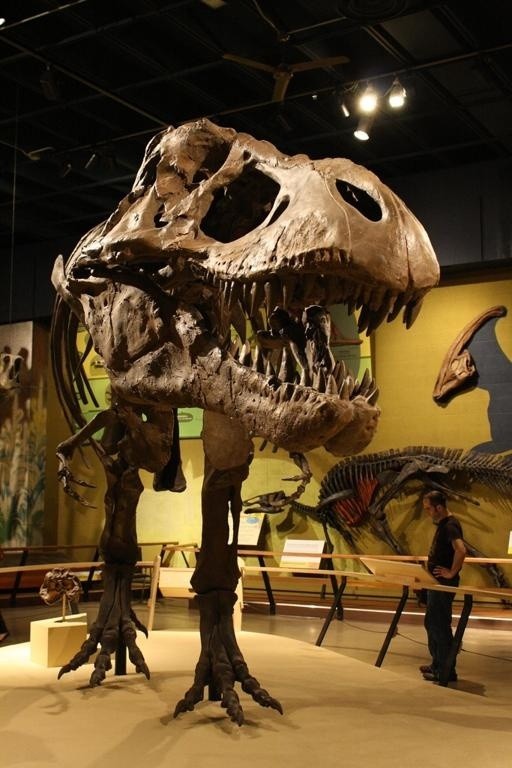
<box><xmin>421</xmin><ymin>664</ymin><xmax>439</xmax><ymax>672</ymax></box>
<box><xmin>424</xmin><ymin>672</ymin><xmax>458</xmax><ymax>681</ymax></box>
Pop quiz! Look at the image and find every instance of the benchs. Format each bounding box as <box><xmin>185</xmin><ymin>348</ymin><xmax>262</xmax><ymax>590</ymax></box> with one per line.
<box><xmin>238</xmin><ymin>548</ymin><xmax>512</xmax><ymax>689</ymax></box>
<box><xmin>1</xmin><ymin>543</ymin><xmax>201</xmax><ymax>640</ymax></box>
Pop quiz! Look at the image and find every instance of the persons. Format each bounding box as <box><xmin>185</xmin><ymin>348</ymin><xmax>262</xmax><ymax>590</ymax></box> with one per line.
<box><xmin>419</xmin><ymin>490</ymin><xmax>465</xmax><ymax>682</ymax></box>
